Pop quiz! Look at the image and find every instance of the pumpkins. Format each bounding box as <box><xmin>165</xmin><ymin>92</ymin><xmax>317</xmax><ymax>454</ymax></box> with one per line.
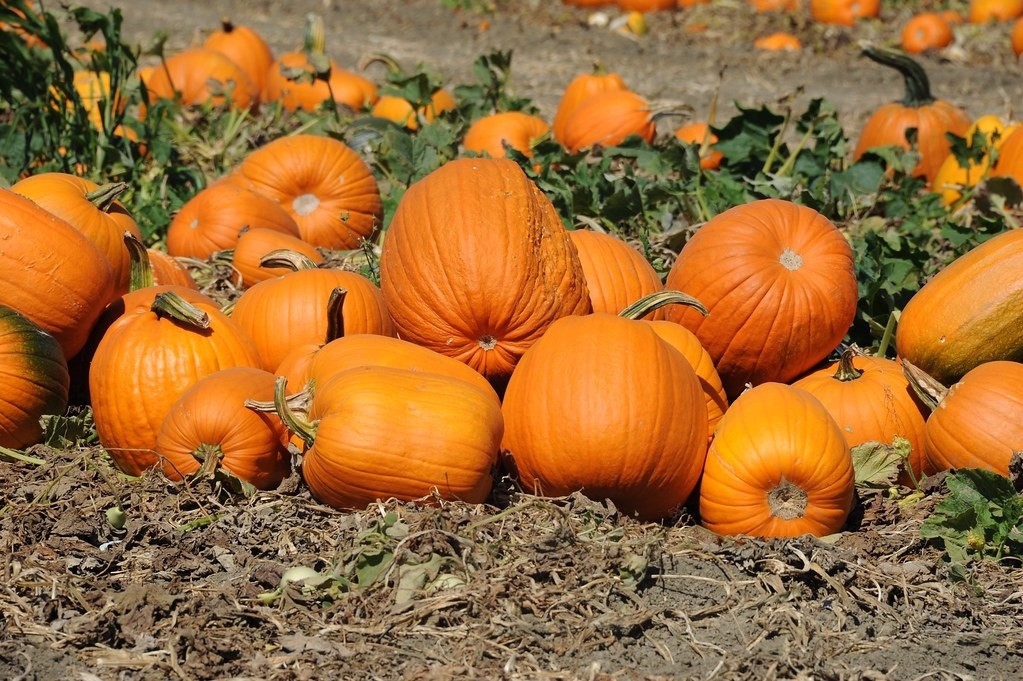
<box><xmin>0</xmin><ymin>0</ymin><xmax>1023</xmax><ymax>539</ymax></box>
